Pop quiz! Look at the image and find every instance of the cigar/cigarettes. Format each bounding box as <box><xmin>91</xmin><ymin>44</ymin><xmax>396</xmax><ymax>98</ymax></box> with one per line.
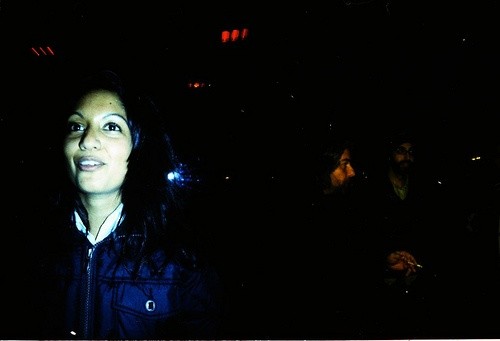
<box><xmin>407</xmin><ymin>262</ymin><xmax>422</xmax><ymax>268</ymax></box>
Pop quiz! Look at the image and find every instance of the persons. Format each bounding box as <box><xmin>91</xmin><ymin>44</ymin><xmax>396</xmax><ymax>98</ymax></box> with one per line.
<box><xmin>272</xmin><ymin>128</ymin><xmax>450</xmax><ymax>340</ymax></box>
<box><xmin>0</xmin><ymin>77</ymin><xmax>221</xmax><ymax>340</ymax></box>
